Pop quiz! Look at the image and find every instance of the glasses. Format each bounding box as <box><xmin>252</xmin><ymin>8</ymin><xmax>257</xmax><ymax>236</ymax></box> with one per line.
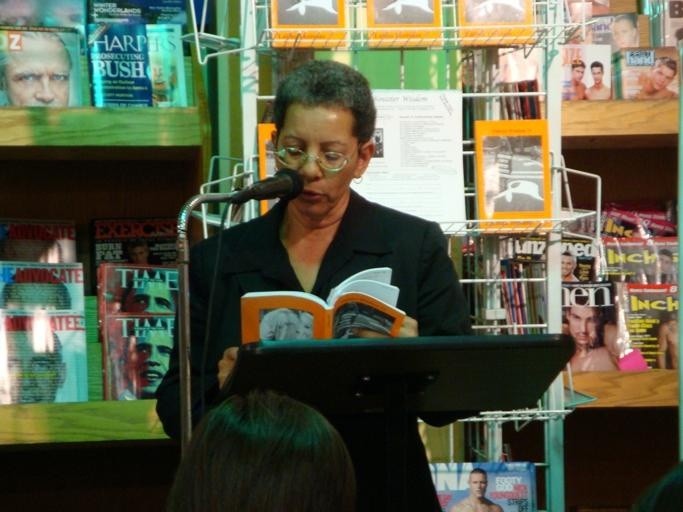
<box><xmin>273</xmin><ymin>145</ymin><xmax>360</xmax><ymax>172</ymax></box>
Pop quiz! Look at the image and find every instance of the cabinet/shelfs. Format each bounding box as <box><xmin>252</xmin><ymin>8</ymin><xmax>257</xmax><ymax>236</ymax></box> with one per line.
<box><xmin>241</xmin><ymin>2</ymin><xmax>683</xmax><ymax>414</ymax></box>
<box><xmin>1</xmin><ymin>0</ymin><xmax>238</xmax><ymax>449</ymax></box>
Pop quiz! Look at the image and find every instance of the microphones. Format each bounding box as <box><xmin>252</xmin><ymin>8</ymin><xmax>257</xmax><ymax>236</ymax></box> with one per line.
<box><xmin>233</xmin><ymin>167</ymin><xmax>304</xmax><ymax>204</ymax></box>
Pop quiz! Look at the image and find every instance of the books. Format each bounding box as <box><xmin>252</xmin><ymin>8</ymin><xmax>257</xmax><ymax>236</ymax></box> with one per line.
<box><xmin>470</xmin><ymin>203</ymin><xmax>678</xmax><ymax>370</ymax></box>
<box><xmin>457</xmin><ymin>0</ymin><xmax>683</xmax><ymax>121</ymax></box>
<box><xmin>474</xmin><ymin>120</ymin><xmax>552</xmax><ymax>230</ymax></box>
<box><xmin>0</xmin><ymin>219</ymin><xmax>179</xmax><ymax>400</ymax></box>
<box><xmin>240</xmin><ymin>268</ymin><xmax>406</xmax><ymax>347</ymax></box>
<box><xmin>1</xmin><ymin>2</ymin><xmax>187</xmax><ymax>108</ymax></box>
<box><xmin>272</xmin><ymin>2</ymin><xmax>348</xmax><ymax>48</ymax></box>
<box><xmin>366</xmin><ymin>2</ymin><xmax>443</xmax><ymax>49</ymax></box>
<box><xmin>430</xmin><ymin>462</ymin><xmax>538</xmax><ymax>511</ymax></box>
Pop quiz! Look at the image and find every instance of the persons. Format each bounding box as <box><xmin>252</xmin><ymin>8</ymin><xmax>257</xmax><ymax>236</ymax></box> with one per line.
<box><xmin>167</xmin><ymin>393</ymin><xmax>355</xmax><ymax>510</ymax></box>
<box><xmin>456</xmin><ymin>468</ymin><xmax>501</xmax><ymax>509</ymax></box>
<box><xmin>155</xmin><ymin>60</ymin><xmax>470</xmax><ymax>512</ymax></box>
